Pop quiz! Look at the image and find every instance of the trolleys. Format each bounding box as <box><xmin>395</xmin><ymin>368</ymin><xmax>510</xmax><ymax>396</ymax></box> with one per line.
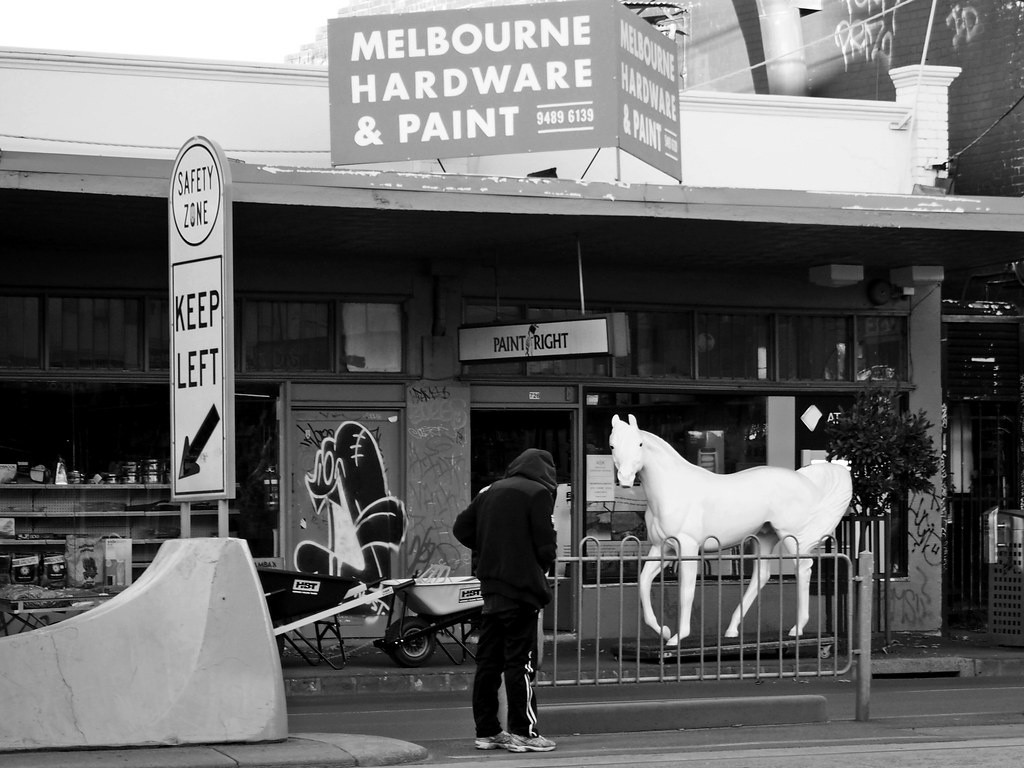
<box><xmin>373</xmin><ymin>568</ymin><xmax>560</xmax><ymax>666</ymax></box>
<box><xmin>256</xmin><ymin>565</ymin><xmax>417</xmax><ymax>672</ymax></box>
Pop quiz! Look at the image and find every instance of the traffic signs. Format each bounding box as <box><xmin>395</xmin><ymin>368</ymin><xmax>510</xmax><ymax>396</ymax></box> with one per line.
<box><xmin>167</xmin><ymin>253</ymin><xmax>235</xmax><ymax>500</ymax></box>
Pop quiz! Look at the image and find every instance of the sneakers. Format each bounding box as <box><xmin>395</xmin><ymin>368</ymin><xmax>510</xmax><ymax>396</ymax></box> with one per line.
<box><xmin>474</xmin><ymin>730</ymin><xmax>510</xmax><ymax>749</ymax></box>
<box><xmin>508</xmin><ymin>734</ymin><xmax>557</xmax><ymax>753</ymax></box>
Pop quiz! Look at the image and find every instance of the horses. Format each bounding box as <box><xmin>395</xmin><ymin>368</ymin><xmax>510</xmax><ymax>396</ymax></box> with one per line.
<box><xmin>609</xmin><ymin>407</ymin><xmax>855</xmax><ymax>661</ymax></box>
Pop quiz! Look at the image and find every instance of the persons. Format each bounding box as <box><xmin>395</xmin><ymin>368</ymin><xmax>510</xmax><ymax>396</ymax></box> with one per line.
<box><xmin>452</xmin><ymin>449</ymin><xmax>558</xmax><ymax>751</ymax></box>
